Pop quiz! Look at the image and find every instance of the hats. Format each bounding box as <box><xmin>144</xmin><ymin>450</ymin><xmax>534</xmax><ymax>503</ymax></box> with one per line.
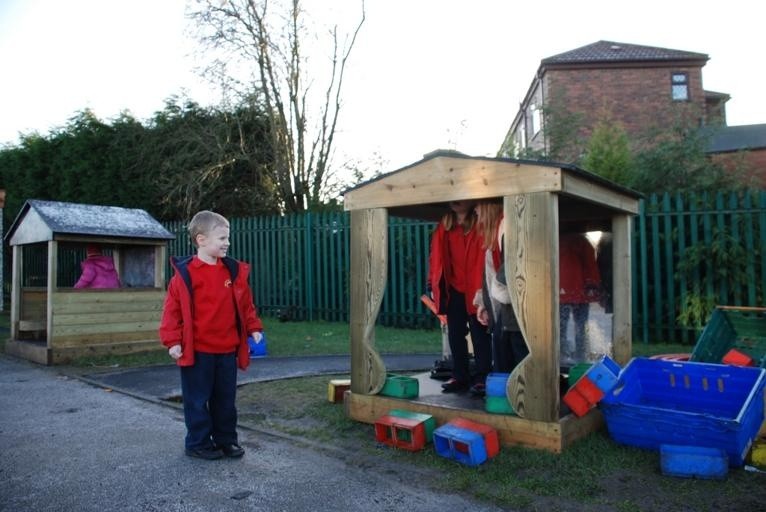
<box><xmin>86</xmin><ymin>244</ymin><xmax>102</xmax><ymax>258</ymax></box>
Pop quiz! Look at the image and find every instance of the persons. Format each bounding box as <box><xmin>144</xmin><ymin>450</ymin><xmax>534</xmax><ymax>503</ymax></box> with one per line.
<box><xmin>70</xmin><ymin>243</ymin><xmax>123</xmax><ymax>289</ymax></box>
<box><xmin>558</xmin><ymin>211</ymin><xmax>602</xmax><ymax>380</ymax></box>
<box><xmin>159</xmin><ymin>209</ymin><xmax>266</xmax><ymax>461</ymax></box>
<box><xmin>428</xmin><ymin>201</ymin><xmax>493</xmax><ymax>398</ymax></box>
<box><xmin>472</xmin><ymin>198</ymin><xmax>528</xmax><ymax>374</ymax></box>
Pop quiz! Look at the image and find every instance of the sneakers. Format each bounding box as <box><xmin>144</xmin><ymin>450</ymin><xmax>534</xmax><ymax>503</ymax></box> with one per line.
<box><xmin>441</xmin><ymin>377</ymin><xmax>472</xmax><ymax>391</ymax></box>
<box><xmin>469</xmin><ymin>382</ymin><xmax>485</xmax><ymax>393</ymax></box>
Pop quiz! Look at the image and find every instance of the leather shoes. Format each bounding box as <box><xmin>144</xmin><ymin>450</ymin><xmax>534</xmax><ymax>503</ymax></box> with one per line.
<box><xmin>184</xmin><ymin>443</ymin><xmax>224</xmax><ymax>460</ymax></box>
<box><xmin>212</xmin><ymin>439</ymin><xmax>245</xmax><ymax>458</ymax></box>
